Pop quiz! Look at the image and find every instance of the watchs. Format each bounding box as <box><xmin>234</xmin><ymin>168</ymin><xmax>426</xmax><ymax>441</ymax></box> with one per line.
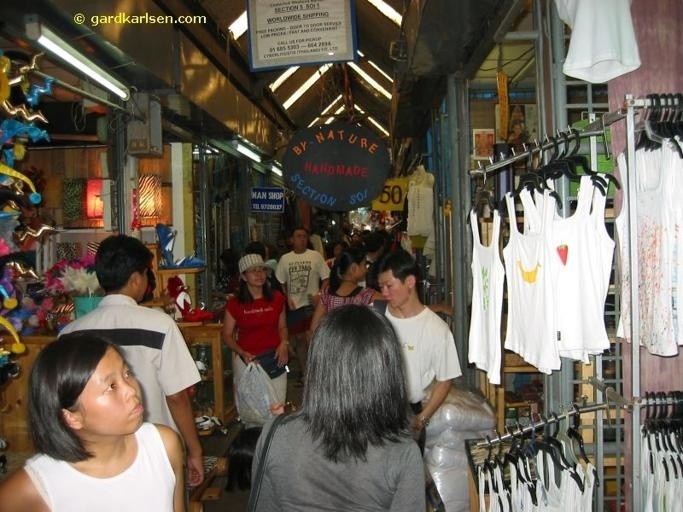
<box><xmin>416</xmin><ymin>413</ymin><xmax>429</xmax><ymax>427</ymax></box>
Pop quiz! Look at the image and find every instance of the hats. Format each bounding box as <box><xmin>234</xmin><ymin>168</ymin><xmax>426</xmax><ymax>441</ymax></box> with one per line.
<box><xmin>238</xmin><ymin>254</ymin><xmax>268</xmax><ymax>272</ymax></box>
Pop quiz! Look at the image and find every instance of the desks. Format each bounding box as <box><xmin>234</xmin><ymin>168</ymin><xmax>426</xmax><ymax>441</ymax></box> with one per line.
<box><xmin>2</xmin><ymin>334</ymin><xmax>62</xmax><ymax>456</ymax></box>
<box><xmin>172</xmin><ymin>323</ymin><xmax>240</xmax><ymax>428</ymax></box>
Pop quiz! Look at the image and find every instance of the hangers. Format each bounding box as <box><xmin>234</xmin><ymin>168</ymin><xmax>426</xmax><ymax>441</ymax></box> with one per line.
<box><xmin>462</xmin><ymin>128</ymin><xmax>622</xmax><ymax>227</ymax></box>
<box><xmin>622</xmin><ymin>91</ymin><xmax>683</xmax><ymax>160</ymax></box>
<box><xmin>641</xmin><ymin>389</ymin><xmax>683</xmax><ymax>482</ymax></box>
<box><xmin>476</xmin><ymin>395</ymin><xmax>601</xmax><ymax>509</ymax></box>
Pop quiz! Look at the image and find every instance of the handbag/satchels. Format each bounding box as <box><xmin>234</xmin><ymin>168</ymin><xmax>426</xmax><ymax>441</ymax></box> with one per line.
<box><xmin>246</xmin><ymin>350</ymin><xmax>291</xmax><ymax>380</ymax></box>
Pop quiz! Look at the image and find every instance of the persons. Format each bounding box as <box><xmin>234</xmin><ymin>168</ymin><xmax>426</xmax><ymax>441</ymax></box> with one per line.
<box><xmin>0</xmin><ymin>330</ymin><xmax>188</xmax><ymax>512</ymax></box>
<box><xmin>370</xmin><ymin>248</ymin><xmax>464</xmax><ymax>458</ymax></box>
<box><xmin>218</xmin><ymin>211</ymin><xmax>453</xmax><ymax>387</ymax></box>
<box><xmin>221</xmin><ymin>254</ymin><xmax>290</xmax><ymax>430</ymax></box>
<box><xmin>58</xmin><ymin>233</ymin><xmax>204</xmax><ymax>488</ymax></box>
<box><xmin>248</xmin><ymin>305</ymin><xmax>428</xmax><ymax>512</ymax></box>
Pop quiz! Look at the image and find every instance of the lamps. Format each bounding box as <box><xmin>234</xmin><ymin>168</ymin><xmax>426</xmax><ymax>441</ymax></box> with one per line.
<box><xmin>228</xmin><ymin>136</ymin><xmax>283</xmax><ymax>181</ymax></box>
<box><xmin>60</xmin><ymin>172</ymin><xmax>173</xmax><ymax>224</ymax></box>
<box><xmin>23</xmin><ymin>13</ymin><xmax>136</xmax><ymax>105</ymax></box>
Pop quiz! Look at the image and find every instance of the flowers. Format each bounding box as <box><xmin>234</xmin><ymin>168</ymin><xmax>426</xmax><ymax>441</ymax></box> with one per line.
<box><xmin>12</xmin><ymin>250</ymin><xmax>103</xmax><ymax>338</ymax></box>
